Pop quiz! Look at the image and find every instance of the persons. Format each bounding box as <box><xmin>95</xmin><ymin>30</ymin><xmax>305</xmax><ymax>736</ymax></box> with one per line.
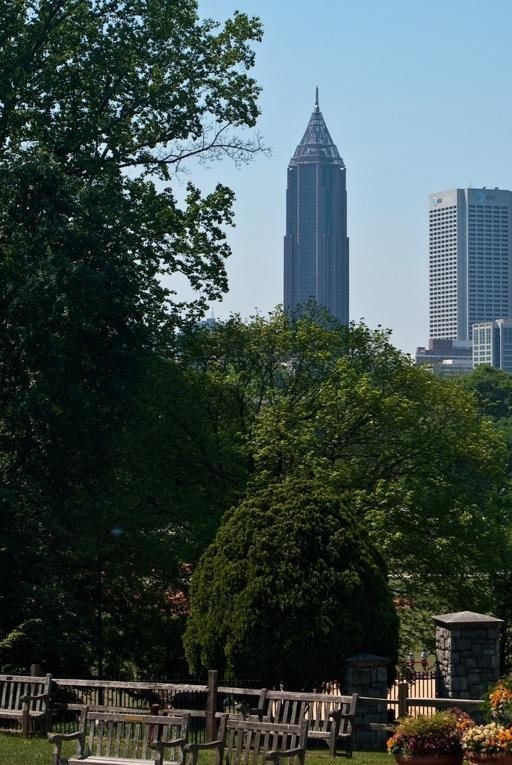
<box><xmin>408</xmin><ymin>652</ymin><xmax>416</xmax><ymax>673</ymax></box>
<box><xmin>420</xmin><ymin>648</ymin><xmax>430</xmax><ymax>672</ymax></box>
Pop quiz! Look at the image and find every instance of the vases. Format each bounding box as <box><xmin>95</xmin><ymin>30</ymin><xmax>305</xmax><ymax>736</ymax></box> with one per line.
<box><xmin>395</xmin><ymin>754</ymin><xmax>462</xmax><ymax>765</ymax></box>
<box><xmin>467</xmin><ymin>751</ymin><xmax>512</xmax><ymax>765</ymax></box>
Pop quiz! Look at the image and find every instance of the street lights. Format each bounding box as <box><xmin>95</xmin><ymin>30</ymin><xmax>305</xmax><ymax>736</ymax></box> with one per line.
<box><xmin>97</xmin><ymin>527</ymin><xmax>123</xmax><ymax>704</ymax></box>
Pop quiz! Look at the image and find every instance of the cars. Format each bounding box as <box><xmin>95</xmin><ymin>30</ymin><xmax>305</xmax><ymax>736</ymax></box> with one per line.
<box><xmin>0</xmin><ymin>672</ymin><xmax>84</xmax><ymax>721</ymax></box>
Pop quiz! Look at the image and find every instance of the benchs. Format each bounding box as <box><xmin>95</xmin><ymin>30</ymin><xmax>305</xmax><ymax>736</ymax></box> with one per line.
<box><xmin>0</xmin><ymin>673</ymin><xmax>52</xmax><ymax>740</ymax></box>
<box><xmin>184</xmin><ymin>713</ymin><xmax>310</xmax><ymax>765</ymax></box>
<box><xmin>251</xmin><ymin>687</ymin><xmax>358</xmax><ymax>760</ymax></box>
<box><xmin>47</xmin><ymin>705</ymin><xmax>191</xmax><ymax>765</ymax></box>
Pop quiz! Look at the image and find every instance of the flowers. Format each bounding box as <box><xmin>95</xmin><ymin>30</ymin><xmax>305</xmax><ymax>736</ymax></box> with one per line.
<box><xmin>459</xmin><ymin>721</ymin><xmax>512</xmax><ymax>761</ymax></box>
<box><xmin>385</xmin><ymin>713</ymin><xmax>468</xmax><ymax>761</ymax></box>
